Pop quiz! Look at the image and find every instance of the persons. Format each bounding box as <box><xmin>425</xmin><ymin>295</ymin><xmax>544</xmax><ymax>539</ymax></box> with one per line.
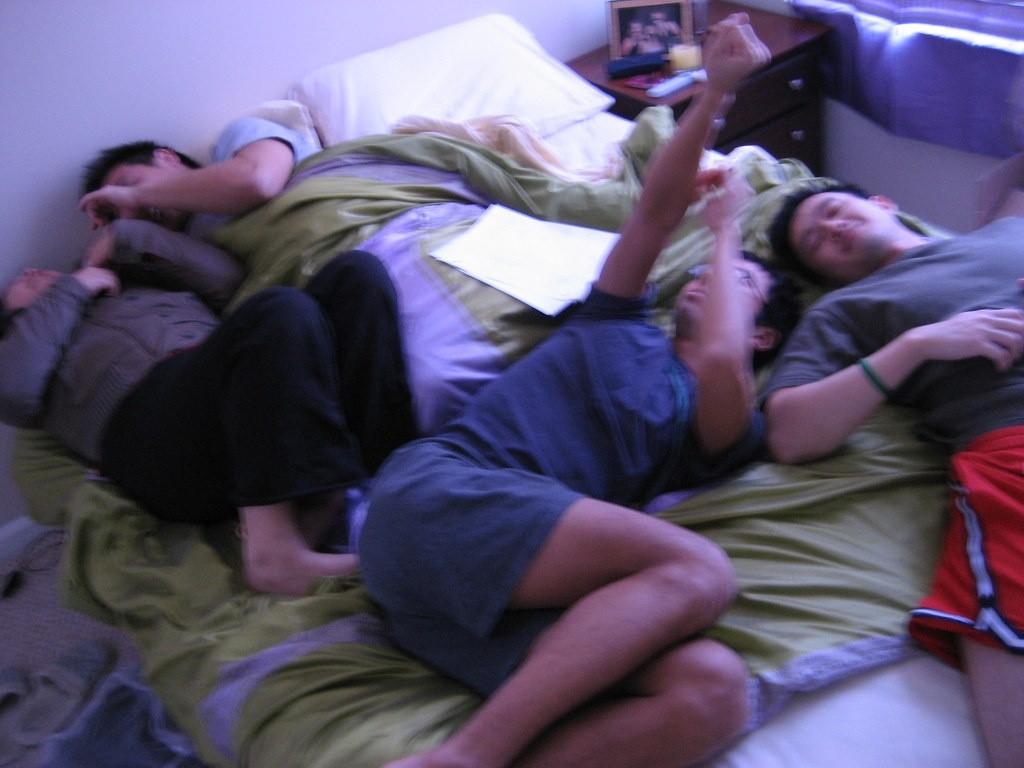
<box><xmin>358</xmin><ymin>14</ymin><xmax>807</xmax><ymax>768</ymax></box>
<box><xmin>0</xmin><ymin>220</ymin><xmax>426</xmax><ymax>597</ymax></box>
<box><xmin>759</xmin><ymin>183</ymin><xmax>1024</xmax><ymax>768</ymax></box>
<box><xmin>77</xmin><ymin>115</ymin><xmax>575</xmax><ymax>431</ymax></box>
<box><xmin>622</xmin><ymin>11</ymin><xmax>681</xmax><ymax>54</ymax></box>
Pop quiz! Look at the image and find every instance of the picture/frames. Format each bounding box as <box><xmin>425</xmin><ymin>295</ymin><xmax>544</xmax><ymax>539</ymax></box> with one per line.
<box><xmin>609</xmin><ymin>0</ymin><xmax>693</xmax><ymax>59</ymax></box>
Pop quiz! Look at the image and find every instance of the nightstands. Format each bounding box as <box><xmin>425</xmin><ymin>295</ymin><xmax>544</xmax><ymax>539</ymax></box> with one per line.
<box><xmin>564</xmin><ymin>0</ymin><xmax>833</xmax><ymax>177</ymax></box>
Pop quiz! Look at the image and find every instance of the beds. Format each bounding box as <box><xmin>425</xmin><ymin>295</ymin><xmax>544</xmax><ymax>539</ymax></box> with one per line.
<box><xmin>0</xmin><ymin>109</ymin><xmax>992</xmax><ymax>767</ymax></box>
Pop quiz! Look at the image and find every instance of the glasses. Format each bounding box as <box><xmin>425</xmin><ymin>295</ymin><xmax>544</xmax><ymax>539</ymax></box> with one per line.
<box><xmin>687</xmin><ymin>263</ymin><xmax>770</xmax><ymax>306</ymax></box>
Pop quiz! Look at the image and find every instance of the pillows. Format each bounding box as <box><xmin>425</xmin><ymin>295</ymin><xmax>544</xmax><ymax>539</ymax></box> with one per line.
<box><xmin>177</xmin><ymin>98</ymin><xmax>323</xmax><ymax>166</ymax></box>
<box><xmin>290</xmin><ymin>11</ymin><xmax>614</xmax><ymax>150</ymax></box>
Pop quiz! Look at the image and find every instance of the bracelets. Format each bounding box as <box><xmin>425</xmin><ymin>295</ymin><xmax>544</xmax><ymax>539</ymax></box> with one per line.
<box><xmin>856</xmin><ymin>359</ymin><xmax>889</xmax><ymax>396</ymax></box>
